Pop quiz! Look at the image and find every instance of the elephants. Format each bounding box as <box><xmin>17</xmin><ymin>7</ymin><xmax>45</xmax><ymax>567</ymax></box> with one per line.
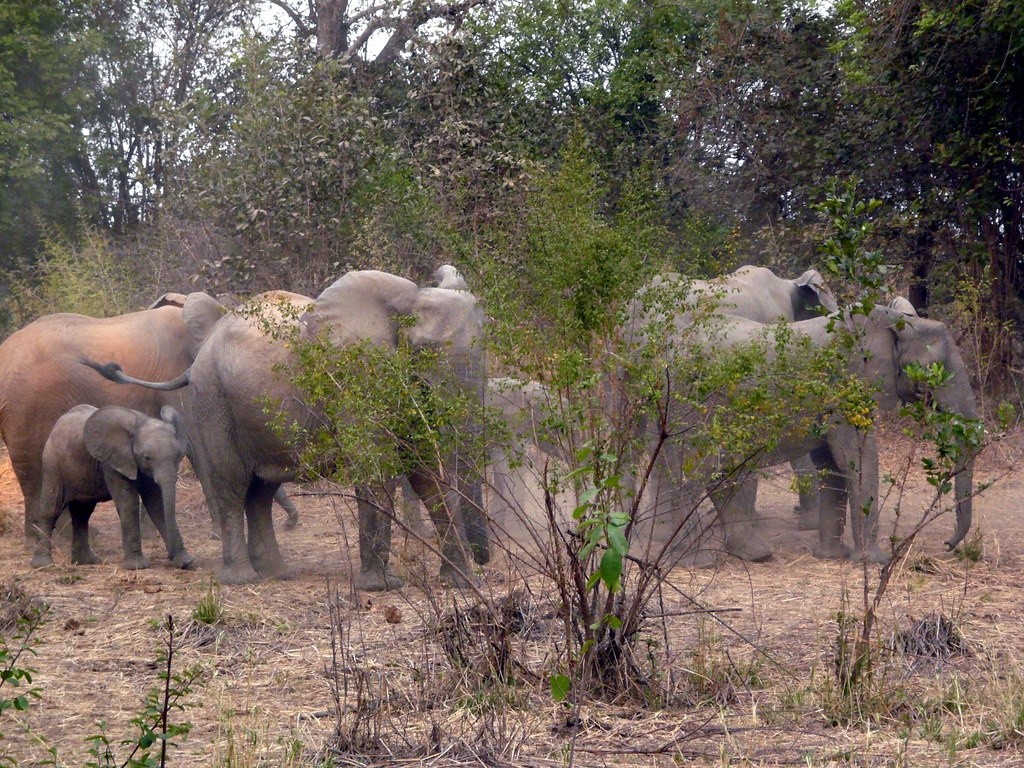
<box><xmin>1</xmin><ymin>264</ymin><xmax>979</xmax><ymax>591</ymax></box>
